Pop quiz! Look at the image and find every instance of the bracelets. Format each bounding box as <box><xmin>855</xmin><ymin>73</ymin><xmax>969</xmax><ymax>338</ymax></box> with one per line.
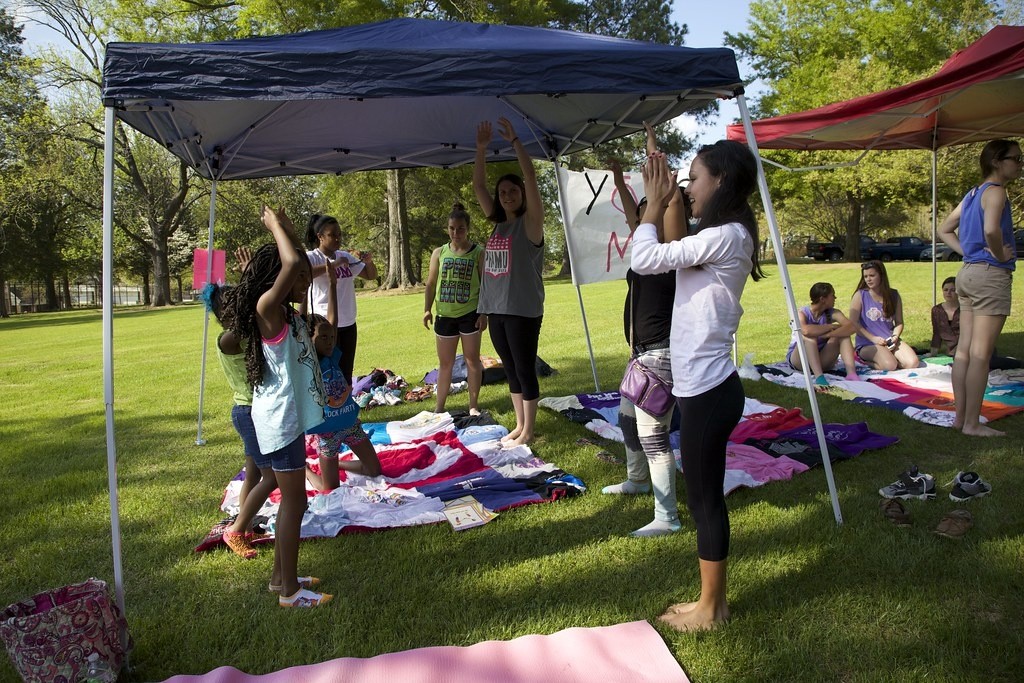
<box><xmin>891</xmin><ymin>334</ymin><xmax>899</xmax><ymax>338</ymax></box>
<box><xmin>511</xmin><ymin>136</ymin><xmax>518</xmax><ymax>143</ymax></box>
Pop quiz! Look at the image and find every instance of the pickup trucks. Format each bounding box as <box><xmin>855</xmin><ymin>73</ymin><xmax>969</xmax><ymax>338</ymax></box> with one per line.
<box><xmin>862</xmin><ymin>237</ymin><xmax>927</xmax><ymax>262</ymax></box>
<box><xmin>805</xmin><ymin>234</ymin><xmax>876</xmax><ymax>261</ymax></box>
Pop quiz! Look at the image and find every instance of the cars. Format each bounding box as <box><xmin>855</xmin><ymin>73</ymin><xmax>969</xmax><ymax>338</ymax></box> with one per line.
<box><xmin>1012</xmin><ymin>229</ymin><xmax>1024</xmax><ymax>258</ymax></box>
<box><xmin>920</xmin><ymin>240</ymin><xmax>963</xmax><ymax>262</ymax></box>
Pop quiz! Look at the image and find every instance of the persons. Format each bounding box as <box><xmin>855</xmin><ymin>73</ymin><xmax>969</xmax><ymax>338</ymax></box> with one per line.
<box><xmin>471</xmin><ymin>116</ymin><xmax>546</xmax><ymax>445</ymax></box>
<box><xmin>423</xmin><ymin>203</ymin><xmax>489</xmax><ymax>417</ymax></box>
<box><xmin>937</xmin><ymin>138</ymin><xmax>1024</xmax><ymax>438</ymax></box>
<box><xmin>785</xmin><ymin>282</ymin><xmax>863</xmax><ymax>385</ymax></box>
<box><xmin>200</xmin><ymin>281</ymin><xmax>279</xmax><ymax>559</ymax></box>
<box><xmin>303</xmin><ymin>211</ymin><xmax>378</xmax><ymax>387</ymax></box>
<box><xmin>849</xmin><ymin>258</ymin><xmax>919</xmax><ymax>371</ymax></box>
<box><xmin>225</xmin><ymin>201</ymin><xmax>334</xmax><ymax>607</ymax></box>
<box><xmin>628</xmin><ymin>140</ymin><xmax>767</xmax><ymax>633</ymax></box>
<box><xmin>930</xmin><ymin>275</ymin><xmax>1024</xmax><ymax>370</ymax></box>
<box><xmin>604</xmin><ymin>127</ymin><xmax>694</xmax><ymax>539</ymax></box>
<box><xmin>305</xmin><ymin>256</ymin><xmax>382</xmax><ymax>493</ymax></box>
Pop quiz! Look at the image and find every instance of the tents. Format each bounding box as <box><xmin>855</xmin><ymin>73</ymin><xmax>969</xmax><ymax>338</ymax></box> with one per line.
<box><xmin>725</xmin><ymin>24</ymin><xmax>1024</xmax><ymax>371</ymax></box>
<box><xmin>101</xmin><ymin>15</ymin><xmax>846</xmax><ymax>672</ymax></box>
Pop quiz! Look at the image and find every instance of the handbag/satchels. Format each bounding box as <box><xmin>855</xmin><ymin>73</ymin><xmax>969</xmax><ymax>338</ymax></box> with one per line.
<box><xmin>619</xmin><ymin>357</ymin><xmax>677</xmax><ymax>417</ymax></box>
<box><xmin>0</xmin><ymin>577</ymin><xmax>132</xmax><ymax>683</ymax></box>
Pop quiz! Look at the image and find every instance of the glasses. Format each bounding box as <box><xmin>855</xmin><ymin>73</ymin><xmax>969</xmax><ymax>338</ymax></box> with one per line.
<box><xmin>860</xmin><ymin>262</ymin><xmax>877</xmax><ymax>268</ymax></box>
<box><xmin>997</xmin><ymin>155</ymin><xmax>1023</xmax><ymax>164</ymax></box>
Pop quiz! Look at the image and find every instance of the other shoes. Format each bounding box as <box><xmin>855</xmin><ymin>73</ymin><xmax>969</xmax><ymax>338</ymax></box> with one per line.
<box><xmin>223</xmin><ymin>530</ymin><xmax>257</xmax><ymax>558</ymax></box>
<box><xmin>245</xmin><ymin>531</ymin><xmax>272</xmax><ymax>545</ymax></box>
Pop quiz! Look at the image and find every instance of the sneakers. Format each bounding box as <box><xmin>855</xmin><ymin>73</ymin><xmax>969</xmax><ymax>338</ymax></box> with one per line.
<box><xmin>878</xmin><ymin>497</ymin><xmax>912</xmax><ymax>528</ymax></box>
<box><xmin>878</xmin><ymin>465</ymin><xmax>936</xmax><ymax>501</ymax></box>
<box><xmin>942</xmin><ymin>470</ymin><xmax>992</xmax><ymax>502</ymax></box>
<box><xmin>936</xmin><ymin>509</ymin><xmax>973</xmax><ymax>539</ymax></box>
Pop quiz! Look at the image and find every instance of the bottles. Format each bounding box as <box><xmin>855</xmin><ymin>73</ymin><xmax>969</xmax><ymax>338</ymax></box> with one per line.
<box><xmin>87</xmin><ymin>653</ymin><xmax>116</xmax><ymax>683</ymax></box>
